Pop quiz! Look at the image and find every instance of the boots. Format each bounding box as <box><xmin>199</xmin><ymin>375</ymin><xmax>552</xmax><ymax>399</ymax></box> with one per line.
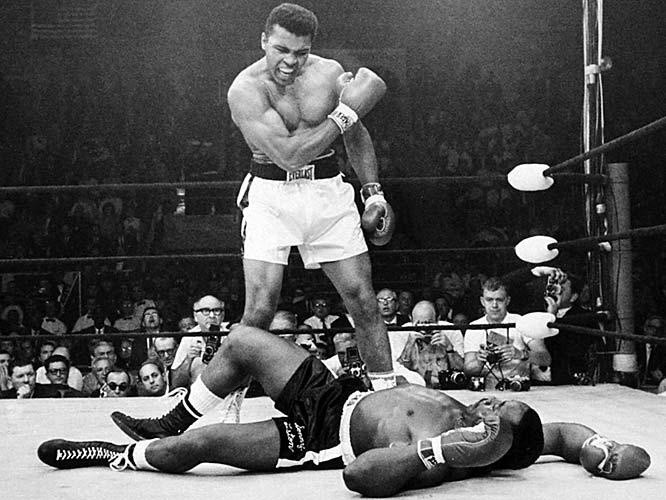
<box><xmin>37</xmin><ymin>438</ymin><xmax>138</xmax><ymax>471</ymax></box>
<box><xmin>215</xmin><ymin>385</ymin><xmax>250</xmax><ymax>424</ymax></box>
<box><xmin>111</xmin><ymin>386</ymin><xmax>202</xmax><ymax>441</ymax></box>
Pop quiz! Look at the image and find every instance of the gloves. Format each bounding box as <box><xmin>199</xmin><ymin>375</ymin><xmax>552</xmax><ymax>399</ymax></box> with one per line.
<box><xmin>359</xmin><ymin>182</ymin><xmax>398</xmax><ymax>245</ymax></box>
<box><xmin>417</xmin><ymin>408</ymin><xmax>514</xmax><ymax>473</ymax></box>
<box><xmin>326</xmin><ymin>66</ymin><xmax>388</xmax><ymax>135</ymax></box>
<box><xmin>578</xmin><ymin>433</ymin><xmax>652</xmax><ymax>481</ymax></box>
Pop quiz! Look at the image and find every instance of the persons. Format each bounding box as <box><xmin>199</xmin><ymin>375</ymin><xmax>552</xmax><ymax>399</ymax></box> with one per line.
<box><xmin>37</xmin><ymin>328</ymin><xmax>651</xmax><ymax>496</ymax></box>
<box><xmin>213</xmin><ymin>3</ymin><xmax>398</xmax><ymax>424</ymax></box>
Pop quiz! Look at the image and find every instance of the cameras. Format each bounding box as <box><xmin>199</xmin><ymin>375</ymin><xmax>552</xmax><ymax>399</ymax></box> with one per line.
<box><xmin>202</xmin><ymin>323</ymin><xmax>220</xmax><ymax>363</ymax></box>
<box><xmin>546</xmin><ymin>275</ymin><xmax>559</xmax><ymax>302</ymax></box>
<box><xmin>486</xmin><ymin>346</ymin><xmax>500</xmax><ymax>364</ymax></box>
<box><xmin>505</xmin><ymin>375</ymin><xmax>531</xmax><ymax>392</ymax></box>
<box><xmin>417</xmin><ymin>321</ymin><xmax>436</xmax><ymax>336</ymax></box>
<box><xmin>438</xmin><ymin>369</ymin><xmax>468</xmax><ymax>387</ymax></box>
<box><xmin>347</xmin><ymin>355</ymin><xmax>363</xmax><ymax>378</ymax></box>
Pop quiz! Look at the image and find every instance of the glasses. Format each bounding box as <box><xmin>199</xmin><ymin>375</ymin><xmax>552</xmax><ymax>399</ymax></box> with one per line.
<box><xmin>378</xmin><ymin>295</ymin><xmax>398</xmax><ymax>303</ymax></box>
<box><xmin>48</xmin><ymin>367</ymin><xmax>68</xmax><ymax>375</ymax></box>
<box><xmin>107</xmin><ymin>381</ymin><xmax>131</xmax><ymax>392</ymax></box>
<box><xmin>194</xmin><ymin>307</ymin><xmax>226</xmax><ymax>316</ymax></box>
<box><xmin>156</xmin><ymin>345</ymin><xmax>177</xmax><ymax>357</ymax></box>
<box><xmin>314</xmin><ymin>302</ymin><xmax>328</xmax><ymax>308</ymax></box>
